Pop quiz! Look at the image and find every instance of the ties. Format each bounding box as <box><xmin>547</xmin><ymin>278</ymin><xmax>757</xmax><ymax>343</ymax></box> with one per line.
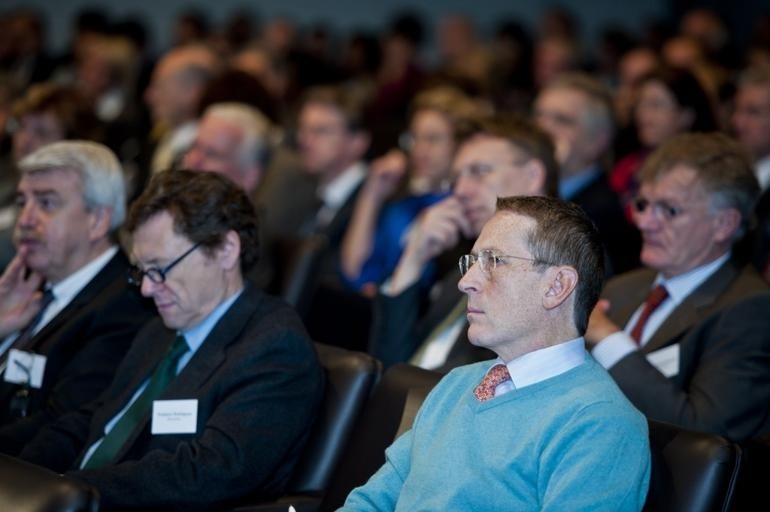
<box><xmin>81</xmin><ymin>331</ymin><xmax>191</xmax><ymax>471</ymax></box>
<box><xmin>472</xmin><ymin>361</ymin><xmax>513</xmax><ymax>404</ymax></box>
<box><xmin>1</xmin><ymin>287</ymin><xmax>55</xmax><ymax>367</ymax></box>
<box><xmin>628</xmin><ymin>285</ymin><xmax>670</xmax><ymax>348</ymax></box>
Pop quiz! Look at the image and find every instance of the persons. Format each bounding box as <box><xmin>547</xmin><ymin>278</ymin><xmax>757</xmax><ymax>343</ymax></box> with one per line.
<box><xmin>0</xmin><ymin>0</ymin><xmax>770</xmax><ymax>512</ymax></box>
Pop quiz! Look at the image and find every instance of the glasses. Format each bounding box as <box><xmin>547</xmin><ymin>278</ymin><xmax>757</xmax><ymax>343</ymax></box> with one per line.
<box><xmin>128</xmin><ymin>240</ymin><xmax>203</xmax><ymax>287</ymax></box>
<box><xmin>632</xmin><ymin>196</ymin><xmax>706</xmax><ymax>219</ymax></box>
<box><xmin>457</xmin><ymin>248</ymin><xmax>559</xmax><ymax>278</ymax></box>
